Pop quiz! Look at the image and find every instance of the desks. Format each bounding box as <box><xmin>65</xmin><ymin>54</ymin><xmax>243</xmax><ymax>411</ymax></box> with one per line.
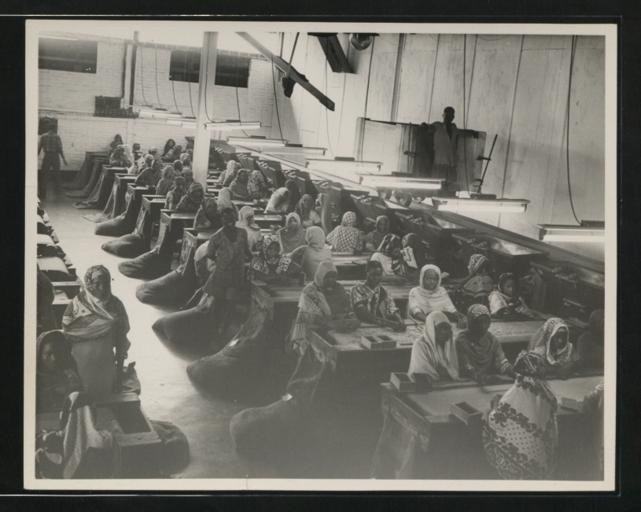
<box><xmin>37</xmin><ymin>145</ymin><xmax>603</xmax><ymax>480</ymax></box>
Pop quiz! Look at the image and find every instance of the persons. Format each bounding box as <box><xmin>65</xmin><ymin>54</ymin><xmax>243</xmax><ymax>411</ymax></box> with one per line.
<box><xmin>421</xmin><ymin>106</ymin><xmax>480</xmax><ymax>178</ymax></box>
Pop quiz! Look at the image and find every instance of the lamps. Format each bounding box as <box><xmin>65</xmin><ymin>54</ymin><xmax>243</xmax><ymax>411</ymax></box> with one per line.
<box><xmin>226</xmin><ymin>34</ymin><xmax>445</xmax><ymax>191</ymax></box>
<box><xmin>431</xmin><ymin>34</ymin><xmax>527</xmax><ymax>215</ymax></box>
<box><xmin>536</xmin><ymin>36</ymin><xmax>604</xmax><ymax>246</ymax></box>
<box><xmin>120</xmin><ymin>33</ymin><xmax>262</xmax><ymax>128</ymax></box>
